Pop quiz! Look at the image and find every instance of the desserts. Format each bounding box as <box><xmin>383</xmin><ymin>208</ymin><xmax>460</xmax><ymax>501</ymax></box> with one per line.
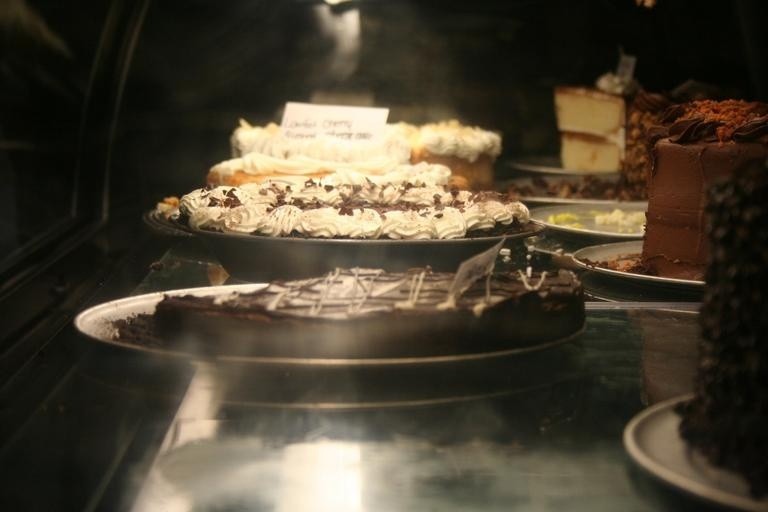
<box><xmin>115</xmin><ymin>266</ymin><xmax>585</xmax><ymax>358</ymax></box>
<box><xmin>157</xmin><ymin>117</ymin><xmax>533</xmax><ymax>238</ymax></box>
<box><xmin>555</xmin><ymin>85</ymin><xmax>768</xmax><ymax>501</ymax></box>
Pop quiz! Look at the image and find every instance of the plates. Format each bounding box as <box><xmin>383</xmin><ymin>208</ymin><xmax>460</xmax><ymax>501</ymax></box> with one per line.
<box><xmin>506</xmin><ymin>157</ymin><xmax>621</xmax><ymax>176</ymax></box>
<box><xmin>143</xmin><ymin>207</ymin><xmax>546</xmax><ymax>282</ymax></box>
<box><xmin>496</xmin><ymin>176</ymin><xmax>650</xmax><ymax>204</ymax></box>
<box><xmin>528</xmin><ymin>203</ymin><xmax>646</xmax><ymax>239</ymax></box>
<box><xmin>74</xmin><ymin>281</ymin><xmax>590</xmax><ymax>368</ymax></box>
<box><xmin>621</xmin><ymin>391</ymin><xmax>768</xmax><ymax>511</ymax></box>
<box><xmin>572</xmin><ymin>239</ymin><xmax>707</xmax><ymax>286</ymax></box>
